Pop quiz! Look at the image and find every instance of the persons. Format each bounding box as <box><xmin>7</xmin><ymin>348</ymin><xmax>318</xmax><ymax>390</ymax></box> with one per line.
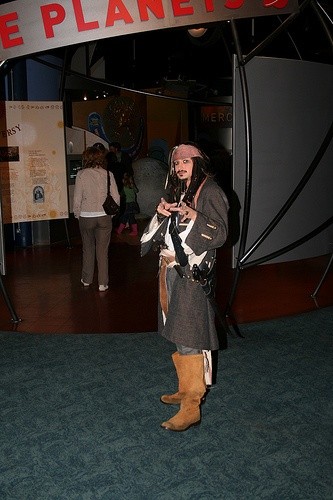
<box><xmin>141</xmin><ymin>144</ymin><xmax>229</xmax><ymax>431</ymax></box>
<box><xmin>73</xmin><ymin>142</ymin><xmax>139</xmax><ymax>292</ymax></box>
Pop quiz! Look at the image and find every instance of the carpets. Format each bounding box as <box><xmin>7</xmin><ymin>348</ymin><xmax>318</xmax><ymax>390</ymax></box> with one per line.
<box><xmin>0</xmin><ymin>306</ymin><xmax>333</xmax><ymax>500</ymax></box>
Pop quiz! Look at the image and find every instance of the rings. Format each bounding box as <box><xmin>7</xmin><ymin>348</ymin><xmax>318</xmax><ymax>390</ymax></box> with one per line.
<box><xmin>185</xmin><ymin>211</ymin><xmax>189</xmax><ymax>215</ymax></box>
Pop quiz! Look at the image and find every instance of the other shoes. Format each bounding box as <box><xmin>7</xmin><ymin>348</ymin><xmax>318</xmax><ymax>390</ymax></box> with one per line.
<box><xmin>81</xmin><ymin>279</ymin><xmax>89</xmax><ymax>287</ymax></box>
<box><xmin>99</xmin><ymin>285</ymin><xmax>108</xmax><ymax>292</ymax></box>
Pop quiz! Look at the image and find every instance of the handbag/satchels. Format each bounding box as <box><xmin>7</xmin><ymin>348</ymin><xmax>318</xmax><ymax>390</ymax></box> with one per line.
<box><xmin>102</xmin><ymin>195</ymin><xmax>120</xmax><ymax>216</ymax></box>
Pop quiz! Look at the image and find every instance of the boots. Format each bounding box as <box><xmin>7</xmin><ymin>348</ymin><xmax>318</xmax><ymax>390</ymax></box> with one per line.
<box><xmin>161</xmin><ymin>350</ymin><xmax>181</xmax><ymax>405</ymax></box>
<box><xmin>127</xmin><ymin>223</ymin><xmax>139</xmax><ymax>236</ymax></box>
<box><xmin>160</xmin><ymin>353</ymin><xmax>207</xmax><ymax>431</ymax></box>
<box><xmin>114</xmin><ymin>223</ymin><xmax>126</xmax><ymax>236</ymax></box>
